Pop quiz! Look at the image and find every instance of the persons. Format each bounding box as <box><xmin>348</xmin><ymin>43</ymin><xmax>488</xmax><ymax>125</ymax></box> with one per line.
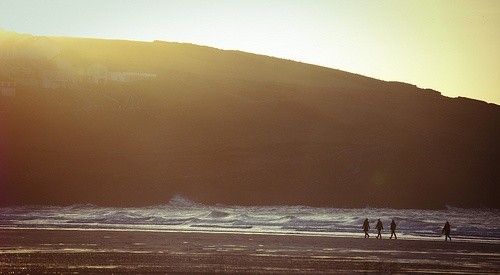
<box><xmin>389</xmin><ymin>220</ymin><xmax>397</xmax><ymax>239</ymax></box>
<box><xmin>441</xmin><ymin>221</ymin><xmax>451</xmax><ymax>242</ymax></box>
<box><xmin>362</xmin><ymin>218</ymin><xmax>370</xmax><ymax>238</ymax></box>
<box><xmin>375</xmin><ymin>219</ymin><xmax>384</xmax><ymax>239</ymax></box>
